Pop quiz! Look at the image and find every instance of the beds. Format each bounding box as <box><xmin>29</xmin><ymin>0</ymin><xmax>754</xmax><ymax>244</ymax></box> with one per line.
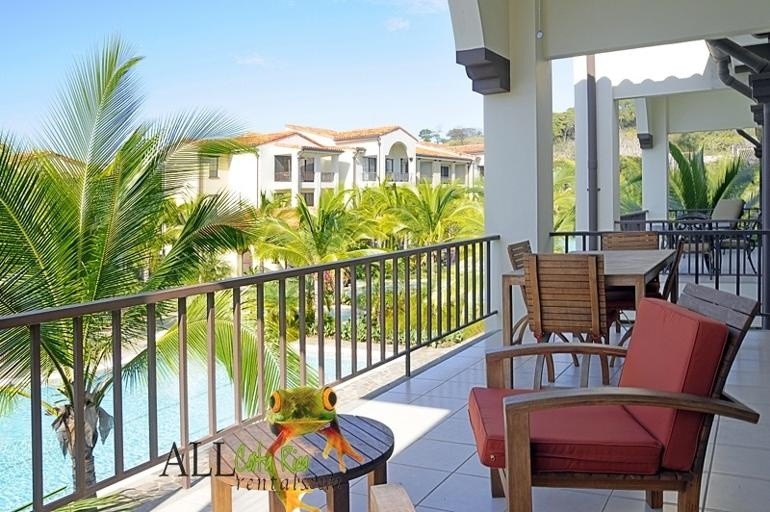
<box><xmin>208</xmin><ymin>414</ymin><xmax>394</xmax><ymax>512</ymax></box>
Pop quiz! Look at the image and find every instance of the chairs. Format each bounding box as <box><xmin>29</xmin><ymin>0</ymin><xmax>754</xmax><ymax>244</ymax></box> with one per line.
<box><xmin>465</xmin><ymin>198</ymin><xmax>762</xmax><ymax>512</ymax></box>
